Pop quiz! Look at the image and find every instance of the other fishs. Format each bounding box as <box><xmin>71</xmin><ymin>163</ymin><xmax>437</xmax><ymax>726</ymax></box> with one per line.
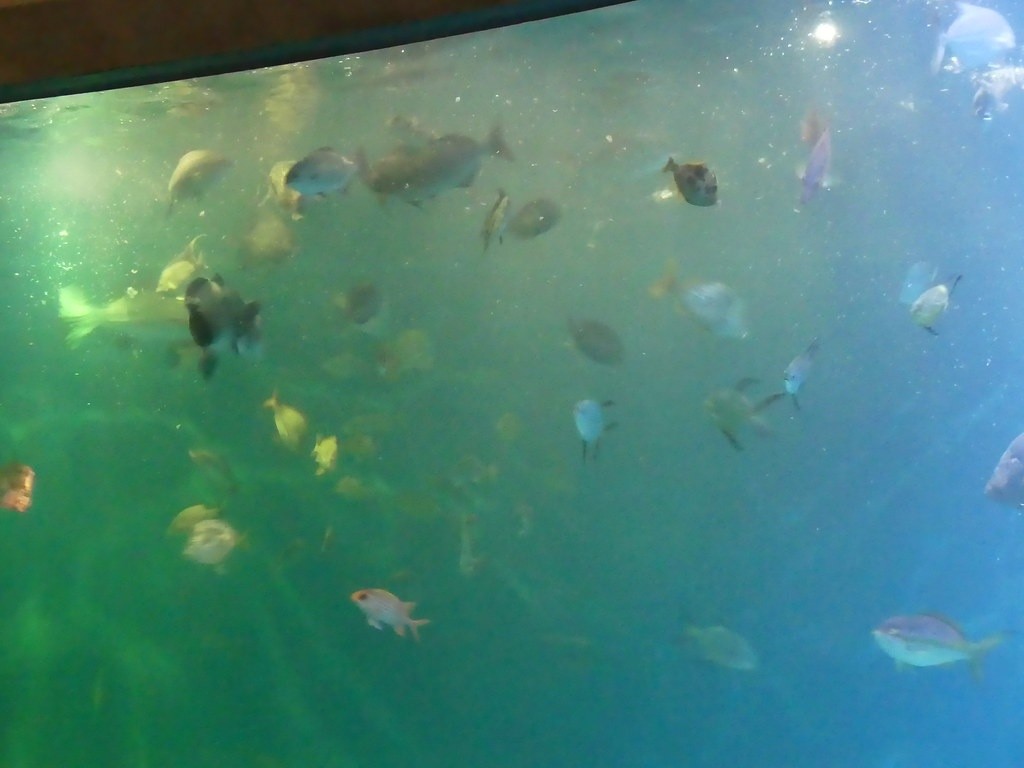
<box><xmin>2</xmin><ymin>0</ymin><xmax>1023</xmax><ymax>679</ymax></box>
